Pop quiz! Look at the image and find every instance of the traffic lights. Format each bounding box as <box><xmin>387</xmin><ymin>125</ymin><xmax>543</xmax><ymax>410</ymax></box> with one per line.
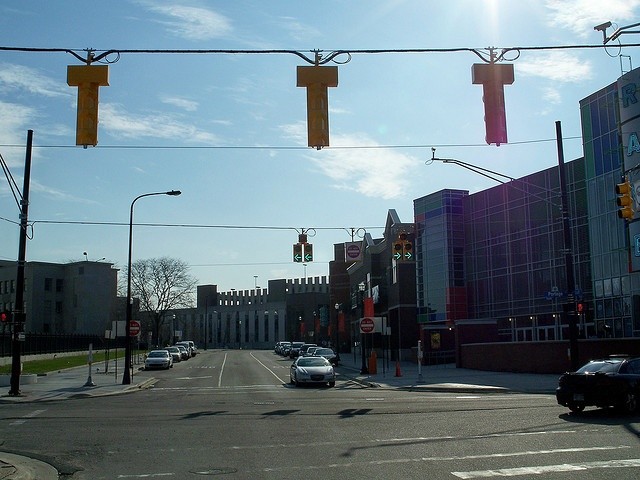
<box><xmin>392</xmin><ymin>232</ymin><xmax>414</xmax><ymax>261</ymax></box>
<box><xmin>577</xmin><ymin>304</ymin><xmax>583</xmax><ymax>314</ymax></box>
<box><xmin>293</xmin><ymin>235</ymin><xmax>312</xmax><ymax>262</ymax></box>
<box><xmin>615</xmin><ymin>181</ymin><xmax>634</xmax><ymax>221</ymax></box>
<box><xmin>0</xmin><ymin>313</ymin><xmax>12</xmax><ymax>322</ymax></box>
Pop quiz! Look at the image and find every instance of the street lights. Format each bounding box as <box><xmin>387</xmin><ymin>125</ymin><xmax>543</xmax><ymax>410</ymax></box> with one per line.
<box><xmin>122</xmin><ymin>189</ymin><xmax>182</xmax><ymax>385</ymax></box>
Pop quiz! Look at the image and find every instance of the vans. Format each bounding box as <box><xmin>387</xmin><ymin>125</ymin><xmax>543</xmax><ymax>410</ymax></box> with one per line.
<box><xmin>175</xmin><ymin>342</ymin><xmax>191</xmax><ymax>358</ymax></box>
<box><xmin>184</xmin><ymin>341</ymin><xmax>196</xmax><ymax>357</ymax></box>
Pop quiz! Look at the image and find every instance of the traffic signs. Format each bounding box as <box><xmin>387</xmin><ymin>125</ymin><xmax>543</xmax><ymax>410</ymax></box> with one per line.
<box><xmin>345</xmin><ymin>241</ymin><xmax>364</xmax><ymax>262</ymax></box>
<box><xmin>359</xmin><ymin>318</ymin><xmax>375</xmax><ymax>333</ymax></box>
<box><xmin>129</xmin><ymin>320</ymin><xmax>141</xmax><ymax>337</ymax></box>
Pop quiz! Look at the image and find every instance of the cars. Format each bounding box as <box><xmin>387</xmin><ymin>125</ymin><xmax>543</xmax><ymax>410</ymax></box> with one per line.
<box><xmin>291</xmin><ymin>356</ymin><xmax>335</xmax><ymax>387</ymax></box>
<box><xmin>173</xmin><ymin>346</ymin><xmax>189</xmax><ymax>360</ymax></box>
<box><xmin>145</xmin><ymin>350</ymin><xmax>173</xmax><ymax>370</ymax></box>
<box><xmin>273</xmin><ymin>341</ymin><xmax>291</xmax><ymax>357</ymax></box>
<box><xmin>306</xmin><ymin>346</ymin><xmax>322</xmax><ymax>357</ymax></box>
<box><xmin>290</xmin><ymin>344</ymin><xmax>303</xmax><ymax>358</ymax></box>
<box><xmin>556</xmin><ymin>354</ymin><xmax>640</xmax><ymax>413</ymax></box>
<box><xmin>164</xmin><ymin>347</ymin><xmax>182</xmax><ymax>362</ymax></box>
<box><xmin>298</xmin><ymin>344</ymin><xmax>317</xmax><ymax>357</ymax></box>
<box><xmin>313</xmin><ymin>348</ymin><xmax>338</xmax><ymax>367</ymax></box>
